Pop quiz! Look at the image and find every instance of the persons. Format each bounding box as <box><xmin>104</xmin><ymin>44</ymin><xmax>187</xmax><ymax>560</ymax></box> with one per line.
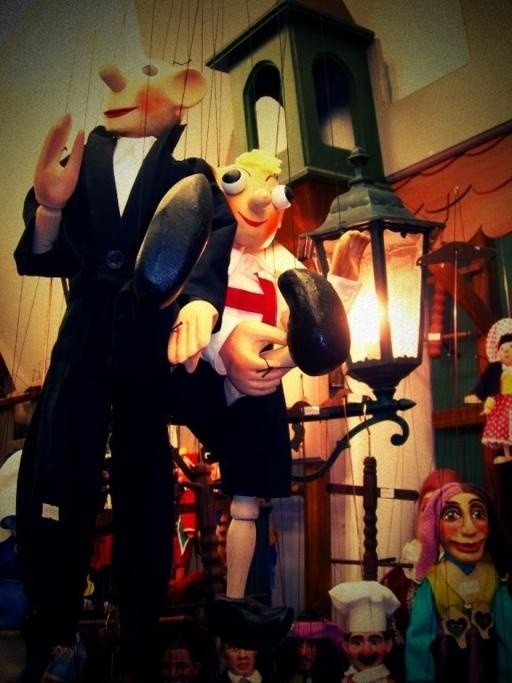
<box><xmin>0</xmin><ymin>57</ymin><xmax>354</xmax><ymax>683</ymax></box>
<box><xmin>329</xmin><ymin>320</ymin><xmax>512</xmax><ymax>683</ymax></box>
<box><xmin>0</xmin><ymin>56</ymin><xmax>512</xmax><ymax>683</ymax></box>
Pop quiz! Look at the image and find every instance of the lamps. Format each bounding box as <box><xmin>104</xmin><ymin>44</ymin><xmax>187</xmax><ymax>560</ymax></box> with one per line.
<box><xmin>287</xmin><ymin>142</ymin><xmax>441</xmax><ymax>478</ymax></box>
